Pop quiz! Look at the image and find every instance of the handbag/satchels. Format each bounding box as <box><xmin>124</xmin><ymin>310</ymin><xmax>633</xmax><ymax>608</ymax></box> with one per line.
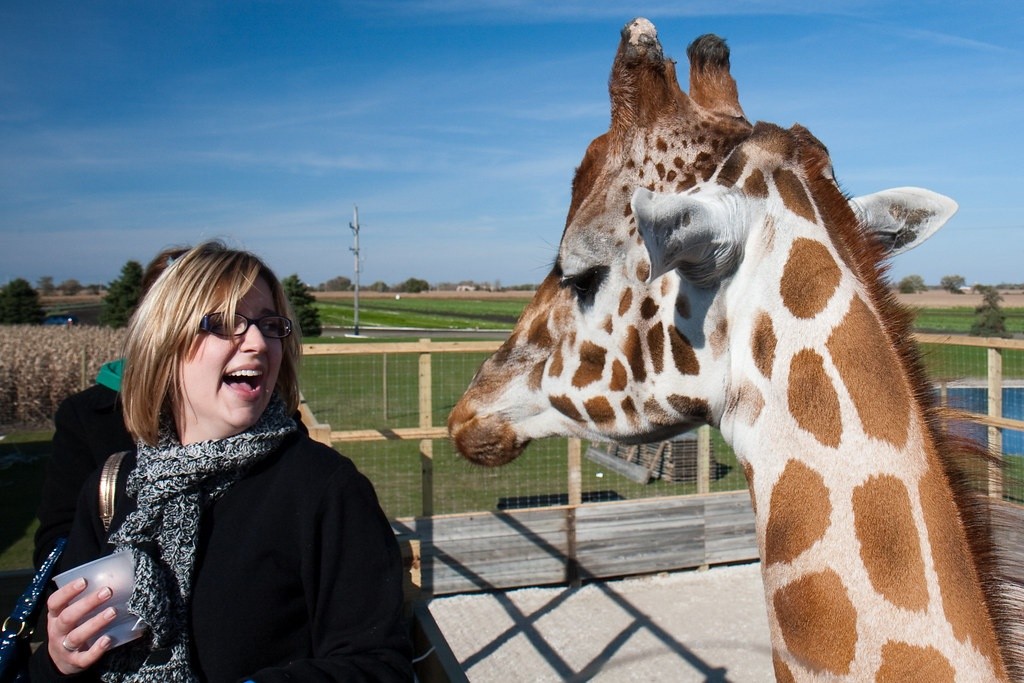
<box><xmin>0</xmin><ymin>539</ymin><xmax>67</xmax><ymax>683</ymax></box>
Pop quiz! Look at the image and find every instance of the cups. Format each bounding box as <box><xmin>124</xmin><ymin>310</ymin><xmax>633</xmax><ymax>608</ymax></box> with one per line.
<box><xmin>52</xmin><ymin>549</ymin><xmax>143</xmax><ymax>653</ymax></box>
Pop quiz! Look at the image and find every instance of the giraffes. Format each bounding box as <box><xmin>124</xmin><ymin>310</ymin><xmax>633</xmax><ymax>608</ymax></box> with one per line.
<box><xmin>445</xmin><ymin>16</ymin><xmax>1024</xmax><ymax>683</ymax></box>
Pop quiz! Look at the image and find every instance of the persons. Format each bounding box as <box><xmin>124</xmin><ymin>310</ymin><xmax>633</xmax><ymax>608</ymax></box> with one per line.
<box><xmin>26</xmin><ymin>238</ymin><xmax>453</xmax><ymax>683</ymax></box>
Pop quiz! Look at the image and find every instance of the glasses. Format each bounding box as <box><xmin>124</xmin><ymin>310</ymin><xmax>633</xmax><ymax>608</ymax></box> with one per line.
<box><xmin>199</xmin><ymin>312</ymin><xmax>292</xmax><ymax>339</ymax></box>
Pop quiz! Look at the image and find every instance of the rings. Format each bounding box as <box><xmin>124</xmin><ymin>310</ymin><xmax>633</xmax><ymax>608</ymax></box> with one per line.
<box><xmin>62</xmin><ymin>640</ymin><xmax>82</xmax><ymax>652</ymax></box>
